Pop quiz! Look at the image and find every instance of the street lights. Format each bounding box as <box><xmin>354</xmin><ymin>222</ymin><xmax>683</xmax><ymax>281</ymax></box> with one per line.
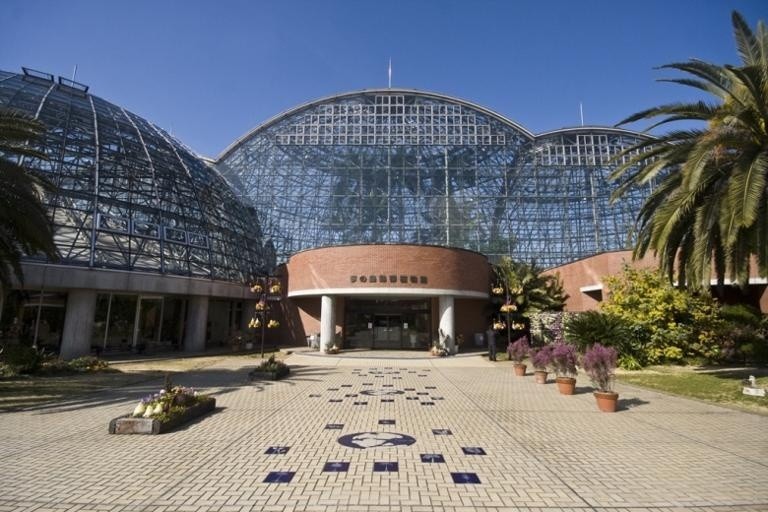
<box><xmin>248</xmin><ymin>275</ymin><xmax>283</xmax><ymax>358</ymax></box>
<box><xmin>492</xmin><ymin>278</ymin><xmax>526</xmax><ymax>360</ymax></box>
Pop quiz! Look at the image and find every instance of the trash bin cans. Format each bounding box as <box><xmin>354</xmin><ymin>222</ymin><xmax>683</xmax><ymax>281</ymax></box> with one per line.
<box><xmin>474</xmin><ymin>333</ymin><xmax>484</xmax><ymax>346</ymax></box>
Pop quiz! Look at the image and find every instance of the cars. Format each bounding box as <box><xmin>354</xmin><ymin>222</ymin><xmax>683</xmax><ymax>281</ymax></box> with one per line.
<box><xmin>372</xmin><ymin>321</ymin><xmax>402</xmax><ymax>342</ymax></box>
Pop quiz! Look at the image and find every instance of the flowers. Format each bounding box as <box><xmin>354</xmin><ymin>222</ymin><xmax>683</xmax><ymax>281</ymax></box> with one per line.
<box><xmin>506</xmin><ymin>334</ymin><xmax>618</xmax><ymax>393</ymax></box>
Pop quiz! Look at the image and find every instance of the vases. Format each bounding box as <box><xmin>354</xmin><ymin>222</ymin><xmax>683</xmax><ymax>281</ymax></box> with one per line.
<box><xmin>593</xmin><ymin>389</ymin><xmax>618</xmax><ymax>413</ymax></box>
